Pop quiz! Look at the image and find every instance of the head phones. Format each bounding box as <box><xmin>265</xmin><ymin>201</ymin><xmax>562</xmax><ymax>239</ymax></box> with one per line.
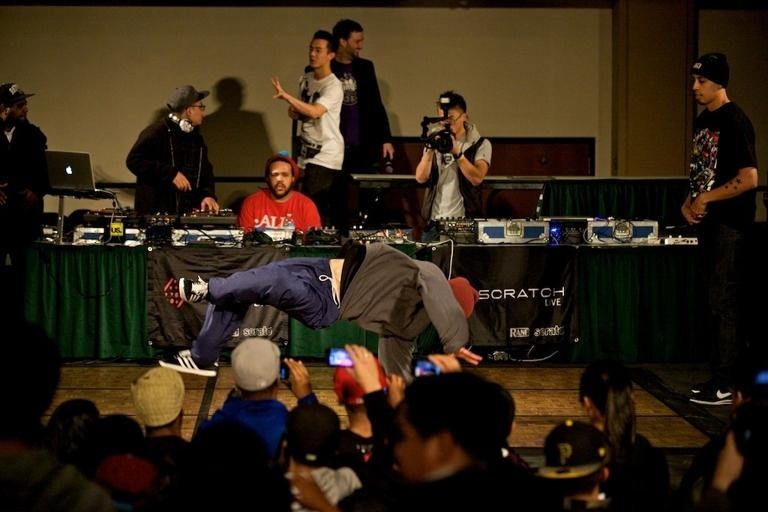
<box><xmin>168</xmin><ymin>111</ymin><xmax>195</xmax><ymax>135</ymax></box>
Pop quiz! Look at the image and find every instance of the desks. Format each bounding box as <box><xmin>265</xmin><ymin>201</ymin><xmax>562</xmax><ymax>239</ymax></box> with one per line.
<box><xmin>536</xmin><ymin>177</ymin><xmax>692</xmax><ymax>221</ymax></box>
<box><xmin>13</xmin><ymin>239</ymin><xmax>704</xmax><ymax>363</ymax></box>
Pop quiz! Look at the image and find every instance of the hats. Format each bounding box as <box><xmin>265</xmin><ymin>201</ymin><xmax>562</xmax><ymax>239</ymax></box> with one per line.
<box><xmin>690</xmin><ymin>53</ymin><xmax>729</xmax><ymax>87</ymax></box>
<box><xmin>285</xmin><ymin>403</ymin><xmax>340</xmax><ymax>467</ymax></box>
<box><xmin>448</xmin><ymin>277</ymin><xmax>479</xmax><ymax>319</ymax></box>
<box><xmin>166</xmin><ymin>85</ymin><xmax>209</xmax><ymax>113</ymax></box>
<box><xmin>538</xmin><ymin>419</ymin><xmax>610</xmax><ymax>479</ymax></box>
<box><xmin>265</xmin><ymin>154</ymin><xmax>299</xmax><ymax>185</ymax></box>
<box><xmin>231</xmin><ymin>337</ymin><xmax>281</xmax><ymax>392</ymax></box>
<box><xmin>0</xmin><ymin>83</ymin><xmax>34</xmax><ymax>102</ymax></box>
<box><xmin>334</xmin><ymin>357</ymin><xmax>387</xmax><ymax>406</ymax></box>
<box><xmin>130</xmin><ymin>366</ymin><xmax>184</xmax><ymax>427</ymax></box>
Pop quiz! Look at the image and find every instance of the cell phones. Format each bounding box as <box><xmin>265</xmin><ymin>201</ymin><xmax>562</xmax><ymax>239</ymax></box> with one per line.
<box><xmin>280</xmin><ymin>364</ymin><xmax>290</xmax><ymax>381</ymax></box>
<box><xmin>324</xmin><ymin>347</ymin><xmax>355</xmax><ymax>368</ymax></box>
<box><xmin>411</xmin><ymin>354</ymin><xmax>439</xmax><ymax>378</ymax></box>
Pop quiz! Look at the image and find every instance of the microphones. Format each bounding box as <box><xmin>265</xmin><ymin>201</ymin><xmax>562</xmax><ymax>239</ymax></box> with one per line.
<box><xmin>383</xmin><ymin>158</ymin><xmax>393</xmax><ymax>174</ymax></box>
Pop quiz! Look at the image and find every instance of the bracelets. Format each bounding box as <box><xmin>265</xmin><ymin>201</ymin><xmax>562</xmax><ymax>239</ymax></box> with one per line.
<box><xmin>453</xmin><ymin>152</ymin><xmax>465</xmax><ymax>161</ymax></box>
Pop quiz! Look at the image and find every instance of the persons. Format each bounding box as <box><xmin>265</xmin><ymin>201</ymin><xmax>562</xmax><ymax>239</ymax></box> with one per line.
<box><xmin>238</xmin><ymin>154</ymin><xmax>323</xmax><ymax>235</ymax></box>
<box><xmin>482</xmin><ymin>375</ymin><xmax>534</xmax><ymax>467</ymax></box>
<box><xmin>196</xmin><ymin>337</ymin><xmax>319</xmax><ymax>462</ymax></box>
<box><xmin>579</xmin><ymin>361</ymin><xmax>668</xmax><ymax>480</ymax></box>
<box><xmin>680</xmin><ymin>52</ymin><xmax>759</xmax><ymax>406</ymax></box>
<box><xmin>536</xmin><ymin>418</ymin><xmax>625</xmax><ymax>509</ymax></box>
<box><xmin>269</xmin><ymin>29</ymin><xmax>345</xmax><ymax>197</ymax></box>
<box><xmin>336</xmin><ymin>372</ymin><xmax>543</xmax><ymax>511</ymax></box>
<box><xmin>696</xmin><ymin>401</ymin><xmax>767</xmax><ymax>512</ymax></box>
<box><xmin>0</xmin><ymin>317</ymin><xmax>130</xmax><ymax>512</ymax></box>
<box><xmin>131</xmin><ymin>360</ymin><xmax>194</xmax><ymax>477</ymax></box>
<box><xmin>416</xmin><ymin>91</ymin><xmax>492</xmax><ymax>244</ymax></box>
<box><xmin>130</xmin><ymin>419</ymin><xmax>160</xmax><ymax>512</ymax></box>
<box><xmin>1</xmin><ymin>83</ymin><xmax>51</xmax><ymax>321</ymax></box>
<box><xmin>333</xmin><ymin>355</ymin><xmax>405</xmax><ymax>460</ymax></box>
<box><xmin>124</xmin><ymin>85</ymin><xmax>219</xmax><ymax>240</ymax></box>
<box><xmin>679</xmin><ymin>367</ymin><xmax>767</xmax><ymax>512</ymax></box>
<box><xmin>155</xmin><ymin>421</ymin><xmax>294</xmax><ymax>512</ymax></box>
<box><xmin>40</xmin><ymin>397</ymin><xmax>130</xmax><ymax>512</ymax></box>
<box><xmin>305</xmin><ymin>20</ymin><xmax>394</xmax><ymax>175</ymax></box>
<box><xmin>279</xmin><ymin>344</ymin><xmax>397</xmax><ymax>512</ymax></box>
<box><xmin>158</xmin><ymin>242</ymin><xmax>484</xmax><ymax>377</ymax></box>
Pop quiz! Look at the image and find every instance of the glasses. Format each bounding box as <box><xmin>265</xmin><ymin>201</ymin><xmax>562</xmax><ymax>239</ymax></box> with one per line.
<box><xmin>190</xmin><ymin>106</ymin><xmax>205</xmax><ymax>111</ymax></box>
<box><xmin>440</xmin><ymin>113</ymin><xmax>463</xmax><ymax>125</ymax></box>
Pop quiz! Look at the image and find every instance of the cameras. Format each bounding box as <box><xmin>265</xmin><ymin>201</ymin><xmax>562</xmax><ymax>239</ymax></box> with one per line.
<box><xmin>420</xmin><ymin>95</ymin><xmax>452</xmax><ymax>156</ymax></box>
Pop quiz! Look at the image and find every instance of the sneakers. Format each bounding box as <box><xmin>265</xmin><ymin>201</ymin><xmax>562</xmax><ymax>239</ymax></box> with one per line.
<box><xmin>690</xmin><ymin>382</ymin><xmax>704</xmax><ymax>394</ymax></box>
<box><xmin>688</xmin><ymin>376</ymin><xmax>734</xmax><ymax>406</ymax></box>
<box><xmin>179</xmin><ymin>275</ymin><xmax>209</xmax><ymax>304</ymax></box>
<box><xmin>158</xmin><ymin>350</ymin><xmax>219</xmax><ymax>377</ymax></box>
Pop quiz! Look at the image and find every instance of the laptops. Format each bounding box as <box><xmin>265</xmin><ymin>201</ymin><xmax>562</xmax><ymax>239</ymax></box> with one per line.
<box><xmin>43</xmin><ymin>151</ymin><xmax>116</xmax><ymax>199</ymax></box>
